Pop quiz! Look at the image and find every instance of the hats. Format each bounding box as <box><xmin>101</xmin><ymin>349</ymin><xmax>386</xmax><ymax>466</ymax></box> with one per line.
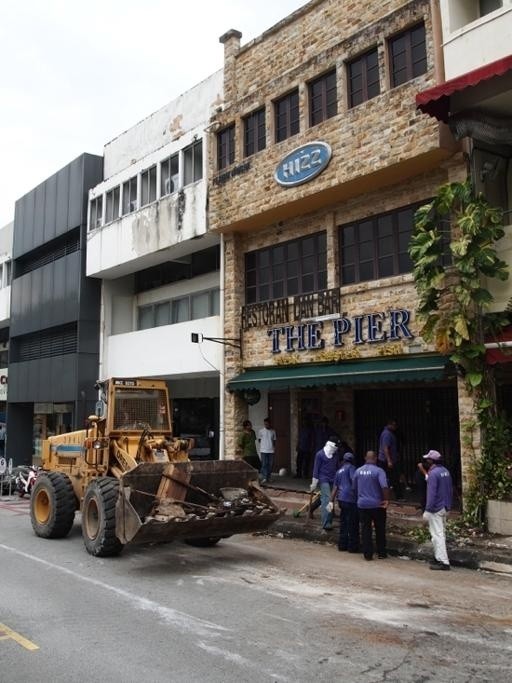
<box><xmin>344</xmin><ymin>453</ymin><xmax>354</xmax><ymax>461</ymax></box>
<box><xmin>423</xmin><ymin>449</ymin><xmax>441</xmax><ymax>461</ymax></box>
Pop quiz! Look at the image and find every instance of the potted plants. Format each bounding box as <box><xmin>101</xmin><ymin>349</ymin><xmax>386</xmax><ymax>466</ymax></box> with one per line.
<box><xmin>462</xmin><ymin>402</ymin><xmax>512</xmax><ymax>536</ymax></box>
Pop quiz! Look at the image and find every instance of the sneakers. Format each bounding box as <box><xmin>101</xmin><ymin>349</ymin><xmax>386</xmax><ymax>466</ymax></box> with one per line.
<box><xmin>429</xmin><ymin>562</ymin><xmax>450</xmax><ymax>570</ymax></box>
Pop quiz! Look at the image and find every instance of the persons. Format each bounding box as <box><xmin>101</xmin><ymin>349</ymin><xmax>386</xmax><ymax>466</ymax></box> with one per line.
<box><xmin>376</xmin><ymin>418</ymin><xmax>409</xmax><ymax>502</ymax></box>
<box><xmin>310</xmin><ymin>436</ymin><xmax>341</xmax><ymax>530</ymax></box>
<box><xmin>294</xmin><ymin>416</ymin><xmax>315</xmax><ymax>480</ymax></box>
<box><xmin>259</xmin><ymin>418</ymin><xmax>278</xmax><ymax>484</ymax></box>
<box><xmin>350</xmin><ymin>449</ymin><xmax>391</xmax><ymax>561</ymax></box>
<box><xmin>416</xmin><ymin>449</ymin><xmax>451</xmax><ymax>571</ymax></box>
<box><xmin>237</xmin><ymin>419</ymin><xmax>262</xmax><ymax>470</ymax></box>
<box><xmin>309</xmin><ymin>416</ymin><xmax>336</xmax><ymax>479</ymax></box>
<box><xmin>326</xmin><ymin>451</ymin><xmax>359</xmax><ymax>553</ymax></box>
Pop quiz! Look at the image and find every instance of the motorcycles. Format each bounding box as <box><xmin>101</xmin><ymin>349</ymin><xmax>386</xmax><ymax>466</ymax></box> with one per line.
<box><xmin>14</xmin><ymin>464</ymin><xmax>38</xmax><ymax>499</ymax></box>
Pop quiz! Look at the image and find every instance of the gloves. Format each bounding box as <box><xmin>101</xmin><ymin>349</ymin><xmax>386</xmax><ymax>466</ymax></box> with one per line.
<box><xmin>310</xmin><ymin>478</ymin><xmax>318</xmax><ymax>492</ymax></box>
<box><xmin>326</xmin><ymin>503</ymin><xmax>333</xmax><ymax>513</ymax></box>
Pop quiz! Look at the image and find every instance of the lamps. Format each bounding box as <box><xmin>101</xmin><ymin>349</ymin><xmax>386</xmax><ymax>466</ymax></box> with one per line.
<box><xmin>189</xmin><ymin>331</ymin><xmax>245</xmax><ymax>361</ymax></box>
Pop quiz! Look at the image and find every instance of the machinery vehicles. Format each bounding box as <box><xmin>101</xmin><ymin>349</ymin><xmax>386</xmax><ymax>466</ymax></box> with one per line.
<box><xmin>22</xmin><ymin>374</ymin><xmax>290</xmax><ymax>558</ymax></box>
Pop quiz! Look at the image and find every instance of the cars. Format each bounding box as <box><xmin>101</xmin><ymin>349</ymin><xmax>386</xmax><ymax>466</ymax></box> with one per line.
<box><xmin>181</xmin><ymin>434</ymin><xmax>211</xmax><ymax>457</ymax></box>
